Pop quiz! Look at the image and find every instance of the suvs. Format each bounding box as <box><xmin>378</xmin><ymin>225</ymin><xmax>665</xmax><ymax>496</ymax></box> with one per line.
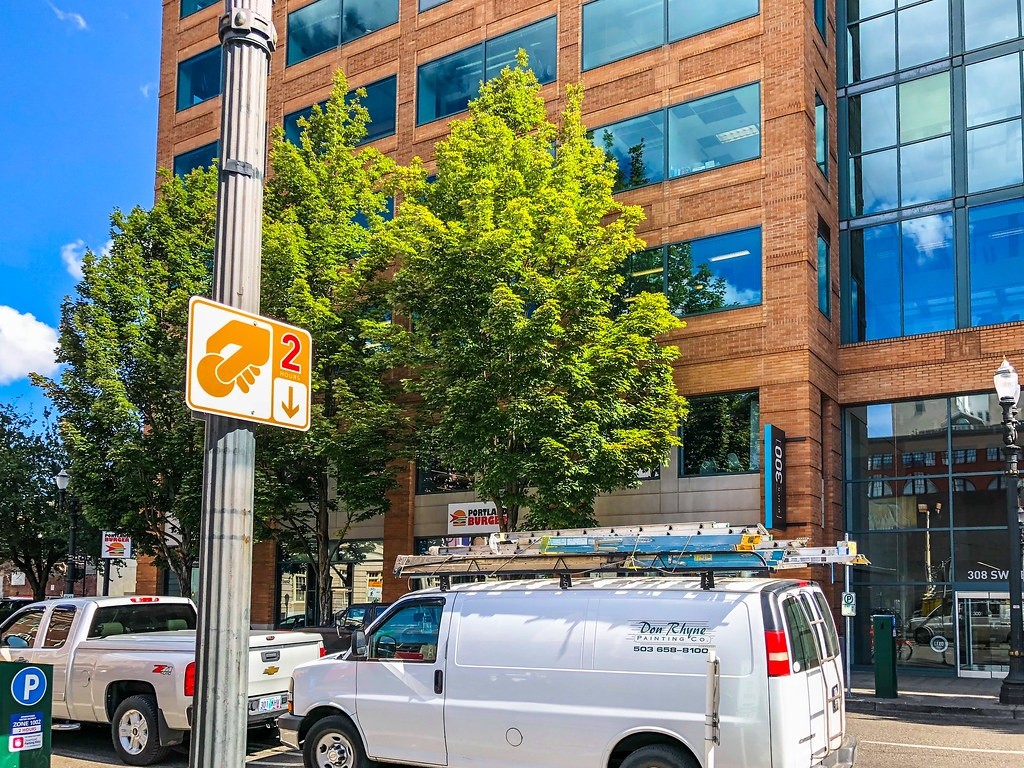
<box><xmin>294</xmin><ymin>604</ymin><xmax>440</xmax><ymax>637</ymax></box>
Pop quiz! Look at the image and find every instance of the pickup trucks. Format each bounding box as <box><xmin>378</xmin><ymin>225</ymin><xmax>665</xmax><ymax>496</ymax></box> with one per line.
<box><xmin>0</xmin><ymin>595</ymin><xmax>325</xmax><ymax>766</ymax></box>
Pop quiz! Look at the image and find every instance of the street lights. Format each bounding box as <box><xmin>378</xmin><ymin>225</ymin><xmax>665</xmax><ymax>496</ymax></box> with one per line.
<box><xmin>918</xmin><ymin>501</ymin><xmax>942</xmax><ymax>598</ymax></box>
<box><xmin>56</xmin><ymin>467</ymin><xmax>78</xmax><ymax>594</ymax></box>
<box><xmin>992</xmin><ymin>352</ymin><xmax>1024</xmax><ymax>704</ymax></box>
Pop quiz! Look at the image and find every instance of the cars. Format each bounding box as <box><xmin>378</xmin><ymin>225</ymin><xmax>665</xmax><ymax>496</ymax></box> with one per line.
<box><xmin>280</xmin><ymin>614</ymin><xmax>306</xmax><ymax>629</ymax></box>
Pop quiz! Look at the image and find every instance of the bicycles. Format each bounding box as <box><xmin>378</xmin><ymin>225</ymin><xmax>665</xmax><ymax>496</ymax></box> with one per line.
<box><xmin>871</xmin><ymin>640</ymin><xmax>913</xmax><ymax>662</ymax></box>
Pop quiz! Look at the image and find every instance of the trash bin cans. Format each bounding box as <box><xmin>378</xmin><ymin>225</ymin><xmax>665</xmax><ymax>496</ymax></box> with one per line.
<box><xmin>873</xmin><ymin>614</ymin><xmax>897</xmax><ymax>698</ymax></box>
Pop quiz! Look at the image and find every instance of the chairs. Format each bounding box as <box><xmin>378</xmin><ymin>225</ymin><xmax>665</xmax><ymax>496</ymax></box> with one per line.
<box><xmin>699</xmin><ymin>461</ymin><xmax>715</xmax><ymax>474</ymax></box>
<box><xmin>727</xmin><ymin>453</ymin><xmax>742</xmax><ymax>468</ymax></box>
<box><xmin>95</xmin><ymin>622</ymin><xmax>123</xmax><ymax>636</ymax></box>
<box><xmin>163</xmin><ymin>619</ymin><xmax>188</xmax><ymax>630</ymax></box>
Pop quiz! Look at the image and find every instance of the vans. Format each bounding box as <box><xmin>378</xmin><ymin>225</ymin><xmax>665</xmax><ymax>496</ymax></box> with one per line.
<box><xmin>0</xmin><ymin>597</ymin><xmax>34</xmax><ymax>622</ymax></box>
<box><xmin>277</xmin><ymin>549</ymin><xmax>858</xmax><ymax>768</ymax></box>
<box><xmin>909</xmin><ymin>591</ymin><xmax>1024</xmax><ymax>646</ymax></box>
